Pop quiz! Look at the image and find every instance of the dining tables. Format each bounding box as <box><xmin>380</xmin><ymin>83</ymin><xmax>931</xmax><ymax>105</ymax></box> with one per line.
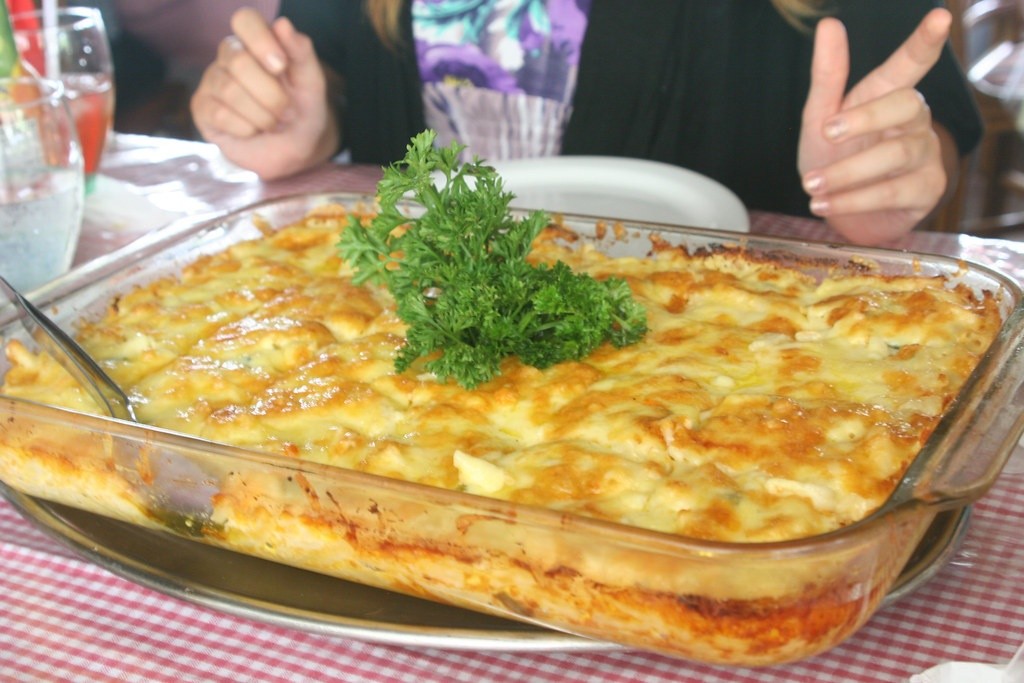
<box><xmin>0</xmin><ymin>132</ymin><xmax>1024</xmax><ymax>682</ymax></box>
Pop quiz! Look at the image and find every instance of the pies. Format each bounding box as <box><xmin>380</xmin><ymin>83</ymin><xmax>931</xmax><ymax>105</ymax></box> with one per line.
<box><xmin>1</xmin><ymin>212</ymin><xmax>1003</xmax><ymax>662</ymax></box>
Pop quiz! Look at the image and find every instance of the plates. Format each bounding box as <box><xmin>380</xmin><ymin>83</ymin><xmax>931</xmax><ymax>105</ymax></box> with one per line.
<box><xmin>0</xmin><ymin>481</ymin><xmax>972</xmax><ymax>656</ymax></box>
<box><xmin>396</xmin><ymin>154</ymin><xmax>752</xmax><ymax>259</ymax></box>
<box><xmin>0</xmin><ymin>190</ymin><xmax>1024</xmax><ymax>668</ymax></box>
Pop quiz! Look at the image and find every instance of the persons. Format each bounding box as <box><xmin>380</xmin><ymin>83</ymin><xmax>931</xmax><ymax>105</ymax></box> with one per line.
<box><xmin>161</xmin><ymin>0</ymin><xmax>991</xmax><ymax>249</ymax></box>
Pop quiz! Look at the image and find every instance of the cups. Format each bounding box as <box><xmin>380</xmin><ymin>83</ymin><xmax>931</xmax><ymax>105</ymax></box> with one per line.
<box><xmin>0</xmin><ymin>76</ymin><xmax>87</xmax><ymax>296</ymax></box>
<box><xmin>5</xmin><ymin>7</ymin><xmax>117</xmax><ymax>175</ymax></box>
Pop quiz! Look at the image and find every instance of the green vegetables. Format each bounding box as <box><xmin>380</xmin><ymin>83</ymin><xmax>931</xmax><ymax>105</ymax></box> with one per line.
<box><xmin>336</xmin><ymin>129</ymin><xmax>647</xmax><ymax>392</ymax></box>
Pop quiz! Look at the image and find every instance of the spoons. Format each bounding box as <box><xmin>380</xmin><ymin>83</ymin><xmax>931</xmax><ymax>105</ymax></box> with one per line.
<box><xmin>0</xmin><ymin>273</ymin><xmax>214</xmax><ymax>522</ymax></box>
